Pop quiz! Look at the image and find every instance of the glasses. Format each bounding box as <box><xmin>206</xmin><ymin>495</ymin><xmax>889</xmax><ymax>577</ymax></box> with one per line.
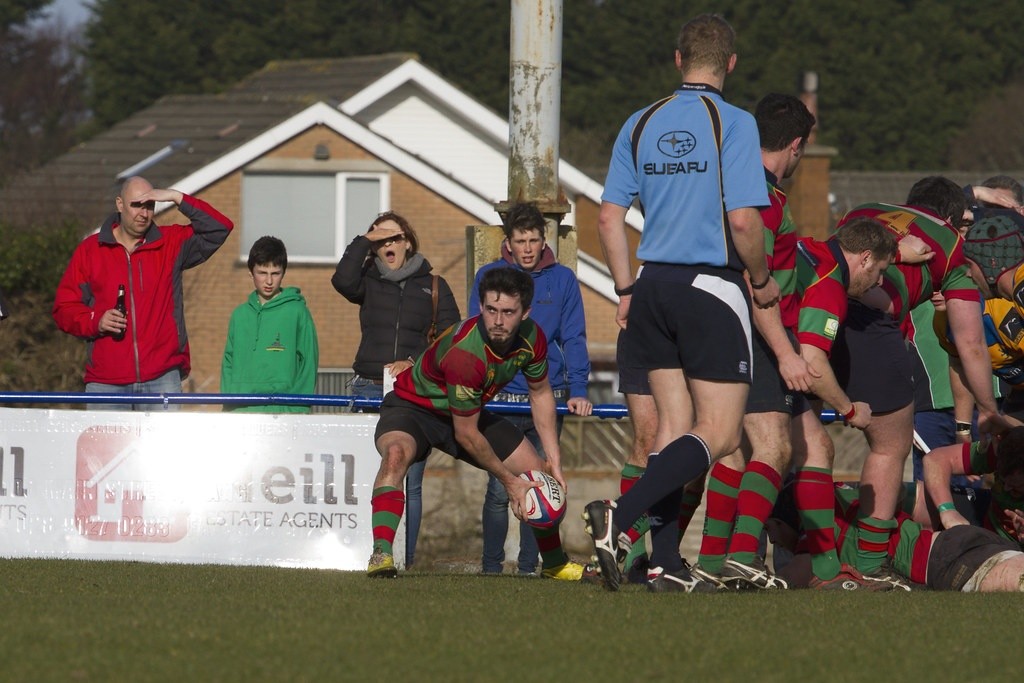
<box><xmin>375</xmin><ymin>235</ymin><xmax>402</xmax><ymax>246</ymax></box>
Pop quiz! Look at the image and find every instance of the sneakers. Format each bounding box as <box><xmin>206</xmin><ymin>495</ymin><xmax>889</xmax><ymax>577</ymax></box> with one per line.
<box><xmin>646</xmin><ymin>559</ymin><xmax>910</xmax><ymax>595</ymax></box>
<box><xmin>616</xmin><ymin>531</ymin><xmax>633</xmax><ymax>585</ymax></box>
<box><xmin>540</xmin><ymin>558</ymin><xmax>599</xmax><ymax>583</ymax></box>
<box><xmin>583</xmin><ymin>500</ymin><xmax>621</xmax><ymax>592</ymax></box>
<box><xmin>366</xmin><ymin>551</ymin><xmax>398</xmax><ymax>578</ymax></box>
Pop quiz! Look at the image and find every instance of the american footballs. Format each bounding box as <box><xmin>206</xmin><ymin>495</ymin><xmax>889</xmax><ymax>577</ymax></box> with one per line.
<box><xmin>515</xmin><ymin>470</ymin><xmax>568</xmax><ymax>530</ymax></box>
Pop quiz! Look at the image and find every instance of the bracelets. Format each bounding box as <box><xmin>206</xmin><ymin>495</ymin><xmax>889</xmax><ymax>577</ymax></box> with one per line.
<box><xmin>954</xmin><ymin>419</ymin><xmax>972</xmax><ymax>436</ymax></box>
<box><xmin>844</xmin><ymin>404</ymin><xmax>855</xmax><ymax>420</ymax></box>
<box><xmin>936</xmin><ymin>502</ymin><xmax>957</xmax><ymax>512</ymax></box>
<box><xmin>896</xmin><ymin>248</ymin><xmax>901</xmax><ymax>264</ymax></box>
<box><xmin>750</xmin><ymin>269</ymin><xmax>771</xmax><ymax>288</ymax></box>
<box><xmin>615</xmin><ymin>283</ymin><xmax>634</xmax><ymax>296</ymax></box>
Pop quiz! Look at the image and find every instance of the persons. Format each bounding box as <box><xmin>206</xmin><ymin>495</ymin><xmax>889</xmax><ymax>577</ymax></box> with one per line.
<box><xmin>583</xmin><ymin>95</ymin><xmax>1024</xmax><ymax>593</ymax></box>
<box><xmin>366</xmin><ymin>266</ymin><xmax>586</xmax><ymax>580</ymax></box>
<box><xmin>220</xmin><ymin>237</ymin><xmax>320</xmax><ymax>414</ymax></box>
<box><xmin>582</xmin><ymin>14</ymin><xmax>783</xmax><ymax>590</ymax></box>
<box><xmin>53</xmin><ymin>175</ymin><xmax>233</xmax><ymax>411</ymax></box>
<box><xmin>331</xmin><ymin>212</ymin><xmax>461</xmax><ymax>415</ymax></box>
<box><xmin>468</xmin><ymin>203</ymin><xmax>592</xmax><ymax>575</ymax></box>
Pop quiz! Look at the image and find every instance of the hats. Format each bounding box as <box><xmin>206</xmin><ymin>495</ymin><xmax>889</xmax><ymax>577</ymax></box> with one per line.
<box><xmin>962</xmin><ymin>215</ymin><xmax>1024</xmax><ymax>299</ymax></box>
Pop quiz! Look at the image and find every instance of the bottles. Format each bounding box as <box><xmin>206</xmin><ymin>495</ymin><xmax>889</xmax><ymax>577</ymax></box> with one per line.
<box><xmin>112</xmin><ymin>284</ymin><xmax>126</xmax><ymax>339</ymax></box>
